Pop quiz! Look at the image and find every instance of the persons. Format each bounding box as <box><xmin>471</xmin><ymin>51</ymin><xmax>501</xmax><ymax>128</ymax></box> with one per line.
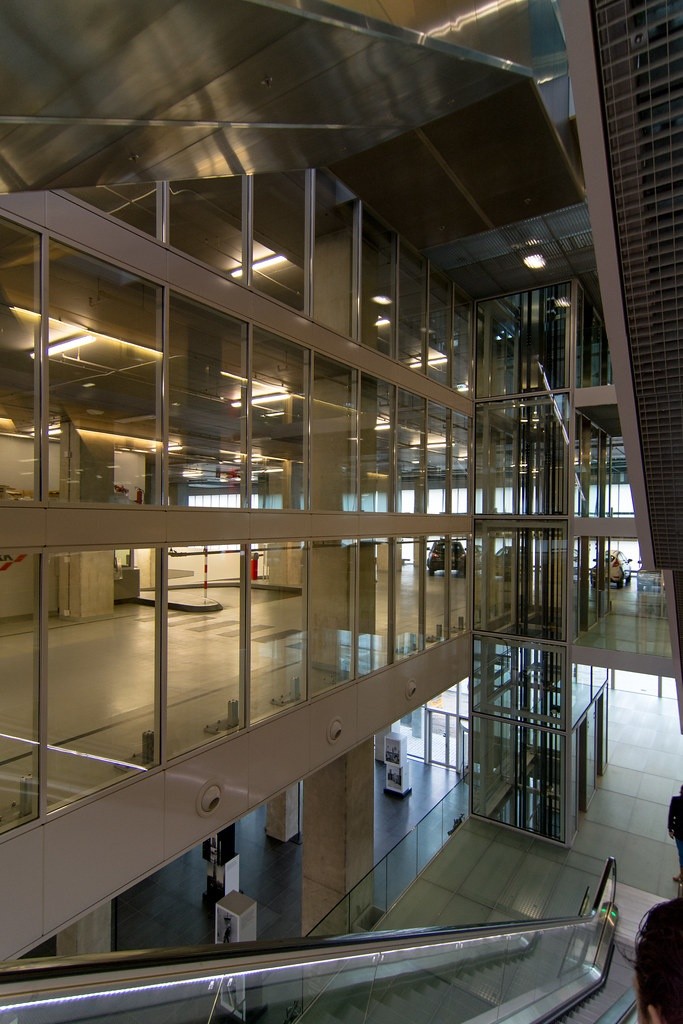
<box><xmin>615</xmin><ymin>895</ymin><xmax>683</xmax><ymax>1024</ymax></box>
<box><xmin>667</xmin><ymin>785</ymin><xmax>683</xmax><ymax>884</ymax></box>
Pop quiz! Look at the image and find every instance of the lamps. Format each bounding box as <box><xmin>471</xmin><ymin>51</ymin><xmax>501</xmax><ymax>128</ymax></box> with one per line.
<box><xmin>228</xmin><ymin>391</ymin><xmax>293</xmax><ymax>408</ymax></box>
<box><xmin>180</xmin><ymin>455</ymin><xmax>285</xmax><ymax>481</ymax></box>
<box><xmin>150</xmin><ymin>445</ymin><xmax>182</xmax><ymax>452</ymax></box>
<box><xmin>30</xmin><ymin>335</ymin><xmax>97</xmax><ymax>359</ymax></box>
<box><xmin>29</xmin><ymin>429</ymin><xmax>61</xmax><ymax>438</ymax></box>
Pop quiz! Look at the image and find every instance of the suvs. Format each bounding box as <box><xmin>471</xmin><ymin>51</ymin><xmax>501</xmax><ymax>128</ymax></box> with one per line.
<box><xmin>605</xmin><ymin>550</ymin><xmax>633</xmax><ymax>588</ymax></box>
<box><xmin>425</xmin><ymin>541</ymin><xmax>467</xmax><ymax>576</ymax></box>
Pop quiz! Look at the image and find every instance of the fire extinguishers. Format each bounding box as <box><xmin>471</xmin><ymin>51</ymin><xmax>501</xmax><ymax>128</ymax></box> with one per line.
<box><xmin>136</xmin><ymin>488</ymin><xmax>144</xmax><ymax>504</ymax></box>
<box><xmin>250</xmin><ymin>557</ymin><xmax>257</xmax><ymax>580</ymax></box>
